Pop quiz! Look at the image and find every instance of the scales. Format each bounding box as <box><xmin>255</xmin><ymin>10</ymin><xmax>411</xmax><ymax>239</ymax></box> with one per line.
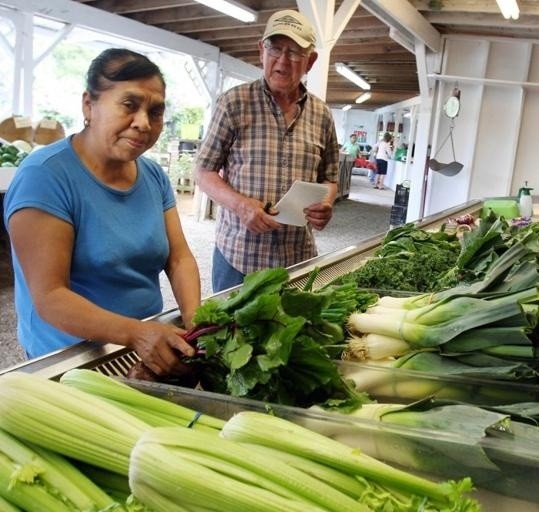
<box><xmin>429</xmin><ymin>81</ymin><xmax>464</xmax><ymax>177</ymax></box>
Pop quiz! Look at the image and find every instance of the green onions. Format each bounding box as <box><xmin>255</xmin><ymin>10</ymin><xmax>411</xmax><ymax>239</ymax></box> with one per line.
<box><xmin>304</xmin><ymin>242</ymin><xmax>539</xmax><ymax>503</ymax></box>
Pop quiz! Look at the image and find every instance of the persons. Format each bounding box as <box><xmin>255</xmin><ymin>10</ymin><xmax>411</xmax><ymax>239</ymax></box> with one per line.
<box><xmin>5</xmin><ymin>47</ymin><xmax>201</xmax><ymax>377</ymax></box>
<box><xmin>366</xmin><ymin>138</ymin><xmax>383</xmax><ymax>187</ymax></box>
<box><xmin>189</xmin><ymin>9</ymin><xmax>340</xmax><ymax>294</ymax></box>
<box><xmin>372</xmin><ymin>132</ymin><xmax>393</xmax><ymax>190</ymax></box>
<box><xmin>340</xmin><ymin>134</ymin><xmax>362</xmax><ymax>167</ymax></box>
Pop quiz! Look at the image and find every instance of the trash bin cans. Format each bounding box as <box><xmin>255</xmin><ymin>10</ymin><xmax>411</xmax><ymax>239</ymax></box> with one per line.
<box><xmin>178</xmin><ymin>139</ymin><xmax>194</xmax><ymax>159</ymax></box>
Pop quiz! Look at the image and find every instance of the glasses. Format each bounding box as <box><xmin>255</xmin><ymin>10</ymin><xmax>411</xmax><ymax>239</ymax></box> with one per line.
<box><xmin>264</xmin><ymin>44</ymin><xmax>310</xmax><ymax>62</ymax></box>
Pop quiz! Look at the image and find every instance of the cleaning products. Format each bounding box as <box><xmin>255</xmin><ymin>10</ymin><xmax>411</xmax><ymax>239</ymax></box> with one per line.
<box><xmin>518</xmin><ymin>181</ymin><xmax>533</xmax><ymax>217</ymax></box>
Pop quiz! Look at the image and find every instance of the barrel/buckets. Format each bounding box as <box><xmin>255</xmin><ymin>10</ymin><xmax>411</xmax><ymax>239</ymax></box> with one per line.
<box><xmin>388</xmin><ymin>204</ymin><xmax>407</xmax><ymax>226</ymax></box>
<box><xmin>395</xmin><ymin>179</ymin><xmax>411</xmax><ymax>205</ymax></box>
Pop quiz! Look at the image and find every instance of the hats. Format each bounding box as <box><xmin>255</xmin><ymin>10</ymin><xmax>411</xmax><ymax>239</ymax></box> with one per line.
<box><xmin>261</xmin><ymin>8</ymin><xmax>317</xmax><ymax>49</ymax></box>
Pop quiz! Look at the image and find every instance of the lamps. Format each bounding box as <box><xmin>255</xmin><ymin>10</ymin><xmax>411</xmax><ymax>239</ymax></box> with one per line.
<box><xmin>331</xmin><ymin>59</ymin><xmax>371</xmax><ymax>112</ymax></box>
<box><xmin>192</xmin><ymin>0</ymin><xmax>260</xmax><ymax>24</ymax></box>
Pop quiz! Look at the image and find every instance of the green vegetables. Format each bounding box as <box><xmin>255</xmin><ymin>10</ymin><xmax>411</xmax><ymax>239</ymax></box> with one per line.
<box><xmin>0</xmin><ymin>369</ymin><xmax>484</xmax><ymax>512</ymax></box>
